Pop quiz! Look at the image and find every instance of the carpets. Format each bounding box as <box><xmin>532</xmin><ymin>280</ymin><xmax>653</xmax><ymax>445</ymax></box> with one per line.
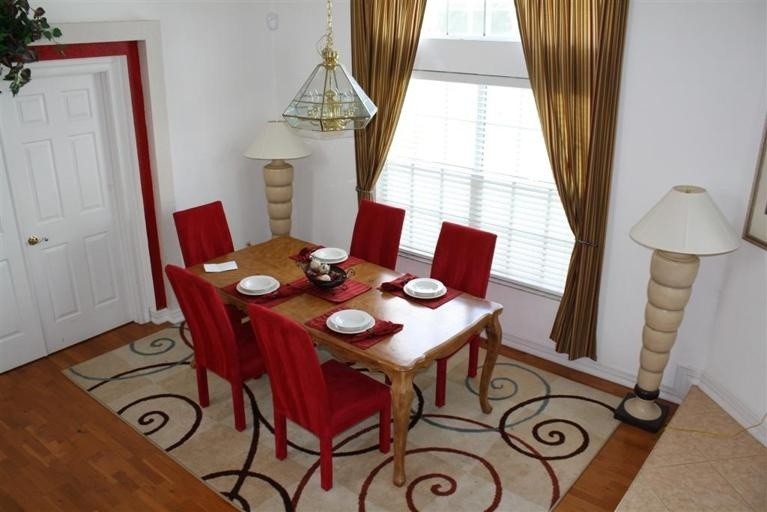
<box><xmin>59</xmin><ymin>317</ymin><xmax>623</xmax><ymax>512</ymax></box>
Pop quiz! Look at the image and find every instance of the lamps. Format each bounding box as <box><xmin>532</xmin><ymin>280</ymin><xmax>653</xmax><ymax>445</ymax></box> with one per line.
<box><xmin>243</xmin><ymin>120</ymin><xmax>312</xmax><ymax>238</ymax></box>
<box><xmin>283</xmin><ymin>1</ymin><xmax>377</xmax><ymax>132</ymax></box>
<box><xmin>612</xmin><ymin>185</ymin><xmax>742</xmax><ymax>434</ymax></box>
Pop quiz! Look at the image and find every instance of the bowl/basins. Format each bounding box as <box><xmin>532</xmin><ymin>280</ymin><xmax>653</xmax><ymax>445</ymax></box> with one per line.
<box><xmin>407</xmin><ymin>278</ymin><xmax>444</xmax><ymax>294</ymax></box>
<box><xmin>315</xmin><ymin>248</ymin><xmax>347</xmax><ymax>260</ymax></box>
<box><xmin>331</xmin><ymin>309</ymin><xmax>371</xmax><ymax>329</ymax></box>
<box><xmin>303</xmin><ymin>263</ymin><xmax>347</xmax><ymax>289</ymax></box>
<box><xmin>240</xmin><ymin>275</ymin><xmax>276</xmax><ymax>292</ymax></box>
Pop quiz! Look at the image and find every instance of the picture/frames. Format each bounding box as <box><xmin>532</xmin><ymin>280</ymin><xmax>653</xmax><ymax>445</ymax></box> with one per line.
<box><xmin>741</xmin><ymin>116</ymin><xmax>767</xmax><ymax>253</ymax></box>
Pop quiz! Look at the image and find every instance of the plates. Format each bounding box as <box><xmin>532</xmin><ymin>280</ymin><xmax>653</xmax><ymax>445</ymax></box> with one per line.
<box><xmin>326</xmin><ymin>313</ymin><xmax>376</xmax><ymax>335</ymax></box>
<box><xmin>403</xmin><ymin>284</ymin><xmax>447</xmax><ymax>300</ymax></box>
<box><xmin>236</xmin><ymin>279</ymin><xmax>281</xmax><ymax>296</ymax></box>
<box><xmin>309</xmin><ymin>253</ymin><xmax>348</xmax><ymax>265</ymax></box>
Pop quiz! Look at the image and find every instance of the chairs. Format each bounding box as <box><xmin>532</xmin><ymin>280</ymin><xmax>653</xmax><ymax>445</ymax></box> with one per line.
<box><xmin>349</xmin><ymin>199</ymin><xmax>406</xmax><ymax>272</ymax></box>
<box><xmin>164</xmin><ymin>263</ymin><xmax>268</xmax><ymax>432</ymax></box>
<box><xmin>172</xmin><ymin>200</ymin><xmax>237</xmax><ymax>268</ymax></box>
<box><xmin>248</xmin><ymin>301</ymin><xmax>392</xmax><ymax>491</ymax></box>
<box><xmin>429</xmin><ymin>221</ymin><xmax>498</xmax><ymax>408</ymax></box>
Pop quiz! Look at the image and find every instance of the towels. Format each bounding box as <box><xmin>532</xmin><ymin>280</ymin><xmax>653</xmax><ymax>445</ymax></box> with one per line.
<box><xmin>257</xmin><ymin>283</ymin><xmax>307</xmax><ymax>303</ymax></box>
<box><xmin>203</xmin><ymin>260</ymin><xmax>238</xmax><ymax>274</ymax></box>
<box><xmin>346</xmin><ymin>321</ymin><xmax>404</xmax><ymax>345</ymax></box>
<box><xmin>380</xmin><ymin>275</ymin><xmax>418</xmax><ymax>293</ymax></box>
<box><xmin>297</xmin><ymin>246</ymin><xmax>316</xmax><ymax>262</ymax></box>
